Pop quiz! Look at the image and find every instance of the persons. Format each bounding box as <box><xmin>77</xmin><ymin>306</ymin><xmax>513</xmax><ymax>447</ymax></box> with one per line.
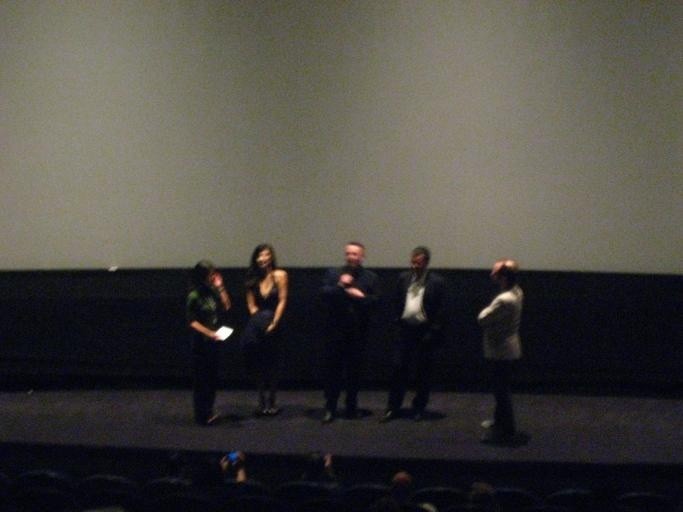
<box><xmin>184</xmin><ymin>260</ymin><xmax>232</xmax><ymax>425</ymax></box>
<box><xmin>477</xmin><ymin>259</ymin><xmax>524</xmax><ymax>446</ymax></box>
<box><xmin>378</xmin><ymin>246</ymin><xmax>450</xmax><ymax>423</ymax></box>
<box><xmin>320</xmin><ymin>242</ymin><xmax>379</xmax><ymax>424</ymax></box>
<box><xmin>245</xmin><ymin>244</ymin><xmax>288</xmax><ymax>415</ymax></box>
<box><xmin>391</xmin><ymin>473</ymin><xmax>437</xmax><ymax>512</ymax></box>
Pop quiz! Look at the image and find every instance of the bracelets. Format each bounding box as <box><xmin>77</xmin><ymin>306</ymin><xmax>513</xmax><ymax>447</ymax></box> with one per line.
<box><xmin>218</xmin><ymin>286</ymin><xmax>225</xmax><ymax>292</ymax></box>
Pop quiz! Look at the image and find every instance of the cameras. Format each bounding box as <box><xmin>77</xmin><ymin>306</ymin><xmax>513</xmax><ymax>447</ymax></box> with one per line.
<box><xmin>228</xmin><ymin>451</ymin><xmax>239</xmax><ymax>464</ymax></box>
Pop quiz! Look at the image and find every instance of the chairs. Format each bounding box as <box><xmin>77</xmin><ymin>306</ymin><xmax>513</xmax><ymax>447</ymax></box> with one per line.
<box><xmin>0</xmin><ymin>470</ymin><xmax>683</xmax><ymax>512</ymax></box>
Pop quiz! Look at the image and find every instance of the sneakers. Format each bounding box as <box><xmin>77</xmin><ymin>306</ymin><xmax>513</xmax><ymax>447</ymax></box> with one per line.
<box><xmin>479</xmin><ymin>420</ymin><xmax>496</xmax><ymax>431</ymax></box>
<box><xmin>255</xmin><ymin>406</ymin><xmax>428</xmax><ymax>423</ymax></box>
